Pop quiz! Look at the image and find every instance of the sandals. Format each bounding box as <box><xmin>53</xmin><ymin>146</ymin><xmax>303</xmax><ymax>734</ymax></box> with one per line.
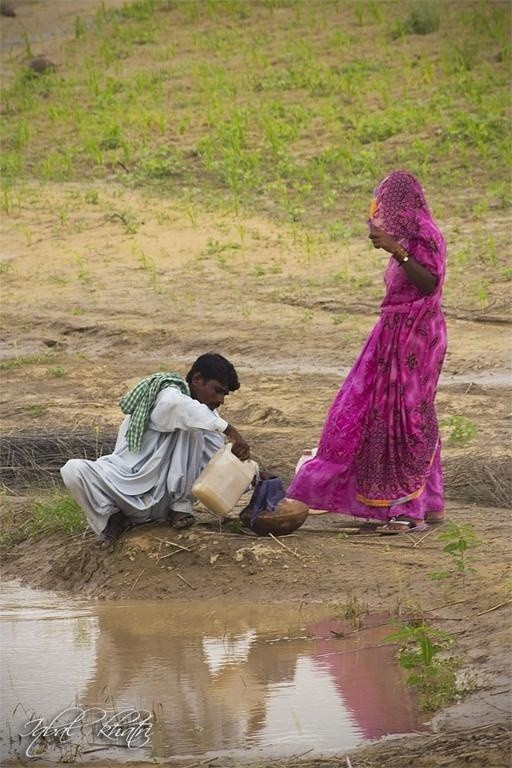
<box><xmin>171</xmin><ymin>514</ymin><xmax>194</xmax><ymax>530</ymax></box>
<box><xmin>374</xmin><ymin>515</ymin><xmax>447</xmax><ymax>533</ymax></box>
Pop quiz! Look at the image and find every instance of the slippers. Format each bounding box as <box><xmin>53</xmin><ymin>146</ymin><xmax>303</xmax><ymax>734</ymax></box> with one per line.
<box><xmin>93</xmin><ymin>516</ymin><xmax>130</xmax><ymax>549</ymax></box>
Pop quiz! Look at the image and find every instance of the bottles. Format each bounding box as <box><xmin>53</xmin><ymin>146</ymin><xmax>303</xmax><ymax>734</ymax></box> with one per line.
<box><xmin>239</xmin><ymin>474</ymin><xmax>308</xmax><ymax>538</ymax></box>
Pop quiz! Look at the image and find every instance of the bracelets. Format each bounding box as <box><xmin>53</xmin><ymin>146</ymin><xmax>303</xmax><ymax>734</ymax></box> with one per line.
<box><xmin>393</xmin><ymin>244</ymin><xmax>409</xmax><ymax>267</ymax></box>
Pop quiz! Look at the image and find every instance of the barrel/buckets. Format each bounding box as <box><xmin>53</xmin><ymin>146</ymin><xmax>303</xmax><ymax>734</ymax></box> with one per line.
<box><xmin>192</xmin><ymin>441</ymin><xmax>257</xmax><ymax>516</ymax></box>
<box><xmin>295</xmin><ymin>448</ymin><xmax>329</xmax><ymax>514</ymax></box>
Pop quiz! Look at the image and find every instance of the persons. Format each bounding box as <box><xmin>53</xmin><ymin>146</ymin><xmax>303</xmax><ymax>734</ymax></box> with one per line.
<box><xmin>285</xmin><ymin>172</ymin><xmax>448</xmax><ymax>534</ymax></box>
<box><xmin>61</xmin><ymin>353</ymin><xmax>275</xmax><ymax>549</ymax></box>
